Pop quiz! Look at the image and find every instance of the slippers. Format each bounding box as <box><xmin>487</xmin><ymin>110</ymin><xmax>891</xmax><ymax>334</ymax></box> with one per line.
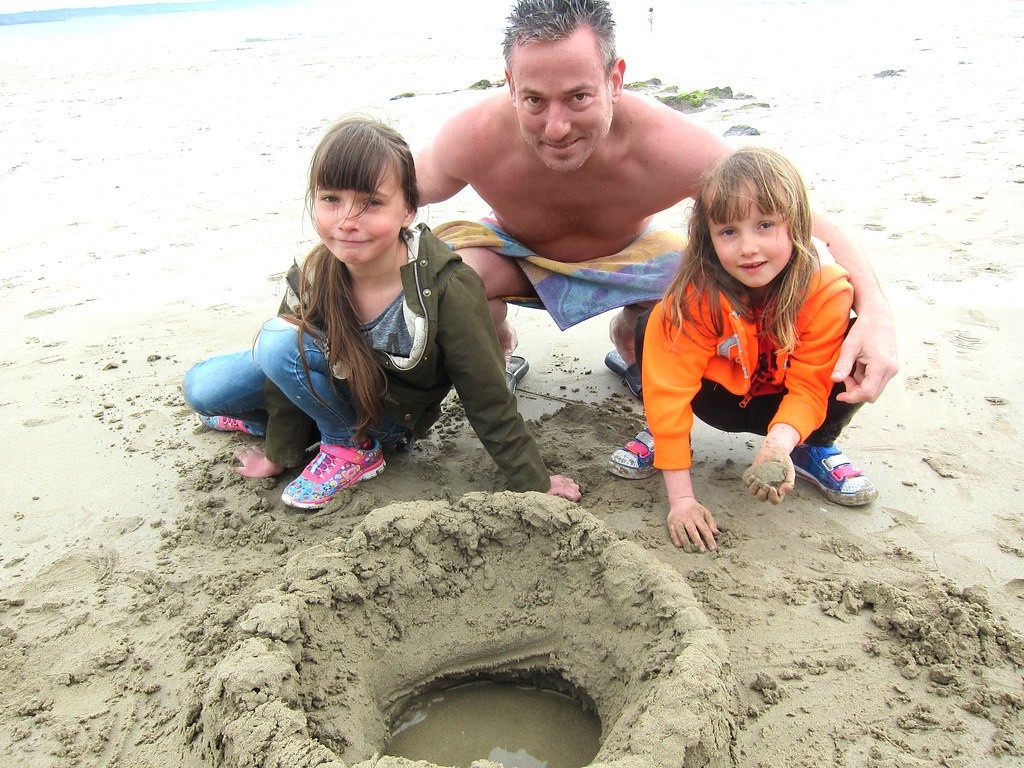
<box><xmin>506</xmin><ymin>356</ymin><xmax>529</xmax><ymax>394</ymax></box>
<box><xmin>605</xmin><ymin>350</ymin><xmax>643</xmax><ymax>402</ymax></box>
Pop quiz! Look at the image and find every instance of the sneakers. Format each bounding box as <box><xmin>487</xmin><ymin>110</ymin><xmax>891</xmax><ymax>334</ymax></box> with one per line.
<box><xmin>789</xmin><ymin>444</ymin><xmax>878</xmax><ymax>506</ymax></box>
<box><xmin>607</xmin><ymin>427</ymin><xmax>694</xmax><ymax>479</ymax></box>
<box><xmin>199</xmin><ymin>414</ymin><xmax>267</xmax><ymax>436</ymax></box>
<box><xmin>281</xmin><ymin>439</ymin><xmax>386</xmax><ymax>509</ymax></box>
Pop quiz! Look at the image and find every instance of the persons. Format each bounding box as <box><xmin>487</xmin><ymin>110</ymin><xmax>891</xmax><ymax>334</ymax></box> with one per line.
<box><xmin>607</xmin><ymin>145</ymin><xmax>879</xmax><ymax>554</ymax></box>
<box><xmin>412</xmin><ymin>0</ymin><xmax>899</xmax><ymax>404</ymax></box>
<box><xmin>181</xmin><ymin>119</ymin><xmax>582</xmax><ymax>511</ymax></box>
